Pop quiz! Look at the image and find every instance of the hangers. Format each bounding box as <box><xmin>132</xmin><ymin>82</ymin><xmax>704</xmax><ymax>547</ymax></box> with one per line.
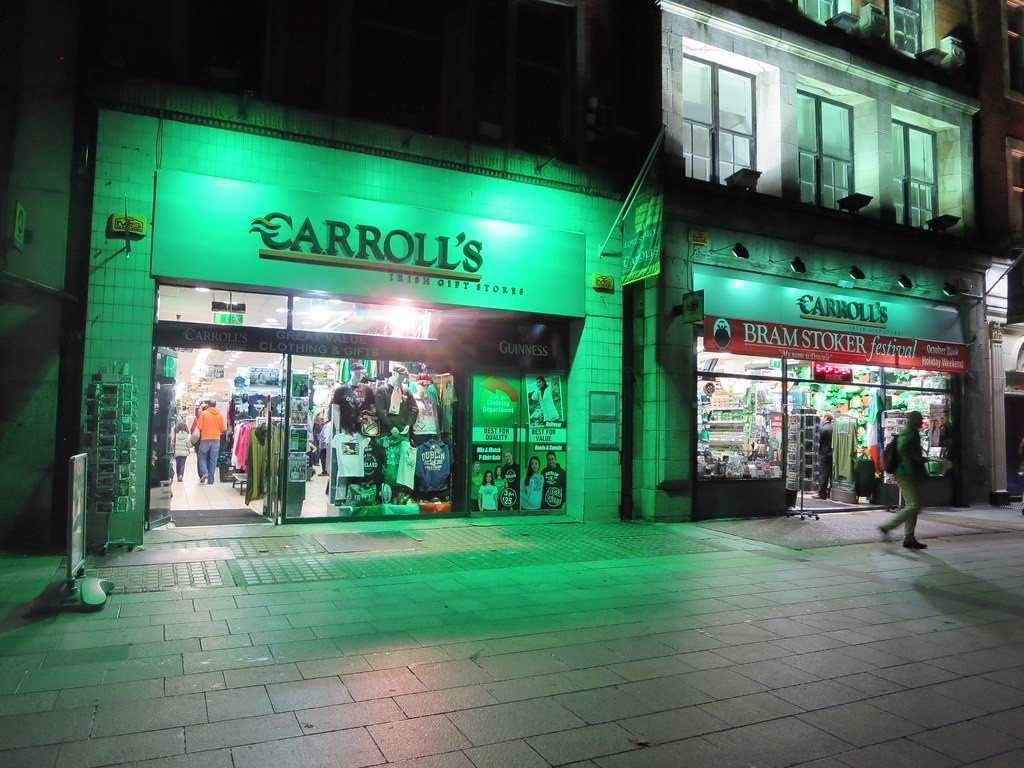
<box><xmin>235</xmin><ymin>419</ymin><xmax>266</xmax><ymax>433</ymax></box>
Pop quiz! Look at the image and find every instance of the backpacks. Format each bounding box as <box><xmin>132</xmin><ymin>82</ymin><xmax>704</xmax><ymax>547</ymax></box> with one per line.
<box><xmin>883</xmin><ymin>431</ymin><xmax>910</xmax><ymax>474</ymax></box>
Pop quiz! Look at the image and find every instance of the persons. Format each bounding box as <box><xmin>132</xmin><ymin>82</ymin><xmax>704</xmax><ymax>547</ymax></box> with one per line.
<box><xmin>412</xmin><ymin>374</ymin><xmax>441</xmax><ymax>446</ymax></box>
<box><xmin>374</xmin><ymin>365</ymin><xmax>419</xmax><ymax>440</ymax></box>
<box><xmin>931</xmin><ymin>420</ymin><xmax>940</xmax><ymax>447</ymax></box>
<box><xmin>519</xmin><ymin>456</ymin><xmax>545</xmax><ymax>510</ymax></box>
<box><xmin>811</xmin><ymin>412</ymin><xmax>834</xmax><ymax>500</ymax></box>
<box><xmin>939</xmin><ymin>417</ymin><xmax>947</xmax><ymax>447</ymax></box>
<box><xmin>541</xmin><ymin>451</ymin><xmax>566</xmax><ymax>509</ymax></box>
<box><xmin>478</xmin><ymin>470</ymin><xmax>498</xmax><ymax>511</ymax></box>
<box><xmin>172</xmin><ymin>424</ymin><xmax>191</xmax><ymax>482</ymax></box>
<box><xmin>197</xmin><ymin>400</ymin><xmax>225</xmax><ymax>484</ymax></box>
<box><xmin>333</xmin><ymin>363</ymin><xmax>376</xmax><ymax>435</ymax></box>
<box><xmin>471</xmin><ymin>462</ymin><xmax>483</xmax><ymax>511</ymax></box>
<box><xmin>878</xmin><ymin>411</ymin><xmax>930</xmax><ymax>549</ymax></box>
<box><xmin>501</xmin><ymin>450</ymin><xmax>519</xmax><ymax>510</ymax></box>
<box><xmin>191</xmin><ymin>406</ymin><xmax>208</xmax><ymax>480</ymax></box>
<box><xmin>494</xmin><ymin>467</ymin><xmax>509</xmax><ymax>508</ymax></box>
<box><xmin>532</xmin><ymin>377</ymin><xmax>559</xmax><ymax>423</ymax></box>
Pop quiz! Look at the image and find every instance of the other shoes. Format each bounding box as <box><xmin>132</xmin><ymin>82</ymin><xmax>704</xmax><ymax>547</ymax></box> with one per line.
<box><xmin>812</xmin><ymin>493</ymin><xmax>827</xmax><ymax>500</ymax></box>
<box><xmin>314</xmin><ymin>463</ymin><xmax>320</xmax><ymax>466</ymax></box>
<box><xmin>177</xmin><ymin>475</ymin><xmax>183</xmax><ymax>482</ymax></box>
<box><xmin>199</xmin><ymin>474</ymin><xmax>208</xmax><ymax>483</ymax></box>
<box><xmin>902</xmin><ymin>538</ymin><xmax>928</xmax><ymax>548</ymax></box>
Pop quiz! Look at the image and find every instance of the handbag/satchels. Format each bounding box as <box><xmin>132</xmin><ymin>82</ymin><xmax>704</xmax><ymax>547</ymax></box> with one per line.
<box><xmin>189</xmin><ymin>425</ymin><xmax>201</xmax><ymax>445</ymax></box>
<box><xmin>346</xmin><ymin>484</ymin><xmax>377</xmax><ymax>506</ymax></box>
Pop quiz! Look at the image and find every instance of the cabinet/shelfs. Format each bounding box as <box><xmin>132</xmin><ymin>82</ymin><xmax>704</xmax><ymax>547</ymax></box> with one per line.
<box><xmin>176</xmin><ymin>394</ymin><xmax>199</xmax><ymax>430</ymax></box>
<box><xmin>711</xmin><ymin>387</ymin><xmax>791</xmax><ymax>454</ymax></box>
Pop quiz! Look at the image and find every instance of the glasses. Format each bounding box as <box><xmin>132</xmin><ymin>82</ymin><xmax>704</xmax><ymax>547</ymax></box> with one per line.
<box><xmin>418</xmin><ymin>382</ymin><xmax>429</xmax><ymax>387</ymax></box>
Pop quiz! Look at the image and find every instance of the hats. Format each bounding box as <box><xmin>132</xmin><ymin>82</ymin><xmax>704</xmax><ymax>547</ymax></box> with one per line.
<box><xmin>415</xmin><ymin>372</ymin><xmax>434</xmax><ymax>384</ymax></box>
<box><xmin>350</xmin><ymin>362</ymin><xmax>365</xmax><ymax>370</ymax></box>
<box><xmin>392</xmin><ymin>363</ymin><xmax>410</xmax><ymax>375</ymax></box>
<box><xmin>234</xmin><ymin>376</ymin><xmax>245</xmax><ymax>387</ymax></box>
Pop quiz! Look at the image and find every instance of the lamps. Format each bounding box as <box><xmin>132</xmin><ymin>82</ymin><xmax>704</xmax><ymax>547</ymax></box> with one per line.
<box><xmin>914</xmin><ymin>48</ymin><xmax>947</xmax><ymax>64</ymax></box>
<box><xmin>920</xmin><ymin>282</ymin><xmax>960</xmax><ymax>297</ymax></box>
<box><xmin>724</xmin><ymin>167</ymin><xmax>763</xmax><ymax>192</ymax></box>
<box><xmin>769</xmin><ymin>256</ymin><xmax>806</xmax><ymax>273</ymax></box>
<box><xmin>825</xmin><ymin>10</ymin><xmax>860</xmax><ymax>31</ymax></box>
<box><xmin>832</xmin><ymin>266</ymin><xmax>865</xmax><ymax>280</ymax></box>
<box><xmin>715</xmin><ymin>241</ymin><xmax>750</xmax><ymax>258</ymax></box>
<box><xmin>873</xmin><ymin>273</ymin><xmax>912</xmax><ymax>289</ymax></box>
<box><xmin>836</xmin><ymin>192</ymin><xmax>874</xmax><ymax>213</ymax></box>
<box><xmin>926</xmin><ymin>214</ymin><xmax>962</xmax><ymax>233</ymax></box>
